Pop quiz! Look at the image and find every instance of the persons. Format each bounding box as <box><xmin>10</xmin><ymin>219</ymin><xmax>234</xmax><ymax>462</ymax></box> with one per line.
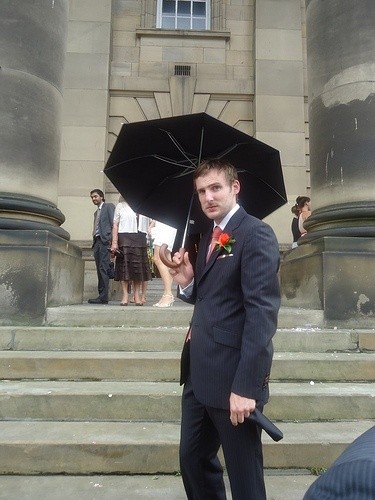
<box><xmin>291</xmin><ymin>196</ymin><xmax>311</xmax><ymax>249</ymax></box>
<box><xmin>168</xmin><ymin>158</ymin><xmax>282</xmax><ymax>500</ymax></box>
<box><xmin>88</xmin><ymin>188</ymin><xmax>177</xmax><ymax>307</ymax></box>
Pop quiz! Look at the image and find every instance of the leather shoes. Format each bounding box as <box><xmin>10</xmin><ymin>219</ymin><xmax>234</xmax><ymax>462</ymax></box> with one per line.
<box><xmin>88</xmin><ymin>296</ymin><xmax>108</xmax><ymax>304</ymax></box>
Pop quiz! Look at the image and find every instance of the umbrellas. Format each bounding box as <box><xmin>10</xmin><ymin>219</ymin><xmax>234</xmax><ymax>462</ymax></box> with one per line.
<box><xmin>101</xmin><ymin>112</ymin><xmax>288</xmax><ymax>268</ymax></box>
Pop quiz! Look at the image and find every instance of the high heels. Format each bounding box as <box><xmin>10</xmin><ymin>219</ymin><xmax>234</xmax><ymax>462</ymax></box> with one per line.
<box><xmin>153</xmin><ymin>294</ymin><xmax>175</xmax><ymax>307</ymax></box>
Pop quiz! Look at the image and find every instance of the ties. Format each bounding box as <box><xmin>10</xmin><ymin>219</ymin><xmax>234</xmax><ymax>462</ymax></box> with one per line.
<box><xmin>96</xmin><ymin>209</ymin><xmax>100</xmax><ymax>237</ymax></box>
<box><xmin>186</xmin><ymin>226</ymin><xmax>222</xmax><ymax>342</ymax></box>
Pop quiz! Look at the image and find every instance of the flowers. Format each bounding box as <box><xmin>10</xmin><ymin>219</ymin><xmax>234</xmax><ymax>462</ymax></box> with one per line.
<box><xmin>213</xmin><ymin>232</ymin><xmax>236</xmax><ymax>253</ymax></box>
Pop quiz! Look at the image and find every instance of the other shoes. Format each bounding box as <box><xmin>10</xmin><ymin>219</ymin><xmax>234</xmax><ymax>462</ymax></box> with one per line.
<box><xmin>120</xmin><ymin>297</ymin><xmax>129</xmax><ymax>306</ymax></box>
<box><xmin>134</xmin><ymin>298</ymin><xmax>143</xmax><ymax>306</ymax></box>
<box><xmin>130</xmin><ymin>295</ymin><xmax>135</xmax><ymax>303</ymax></box>
<box><xmin>141</xmin><ymin>294</ymin><xmax>147</xmax><ymax>303</ymax></box>
